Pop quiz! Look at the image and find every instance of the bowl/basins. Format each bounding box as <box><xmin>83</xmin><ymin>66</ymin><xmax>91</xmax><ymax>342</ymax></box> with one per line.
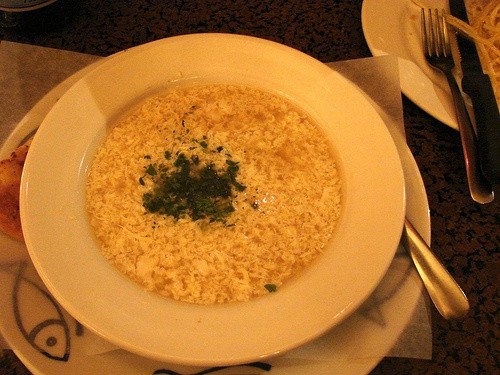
<box><xmin>18</xmin><ymin>34</ymin><xmax>407</xmax><ymax>366</ymax></box>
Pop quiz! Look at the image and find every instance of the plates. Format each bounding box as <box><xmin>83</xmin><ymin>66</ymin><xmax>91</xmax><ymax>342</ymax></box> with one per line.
<box><xmin>362</xmin><ymin>0</ymin><xmax>500</xmax><ymax>137</ymax></box>
<box><xmin>0</xmin><ymin>41</ymin><xmax>430</xmax><ymax>375</ymax></box>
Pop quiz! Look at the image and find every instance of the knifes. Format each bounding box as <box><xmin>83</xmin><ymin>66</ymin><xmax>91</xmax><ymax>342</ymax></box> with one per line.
<box><xmin>447</xmin><ymin>0</ymin><xmax>500</xmax><ymax>182</ymax></box>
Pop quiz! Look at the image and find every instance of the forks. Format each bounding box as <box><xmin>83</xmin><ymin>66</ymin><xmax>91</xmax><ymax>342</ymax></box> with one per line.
<box><xmin>418</xmin><ymin>6</ymin><xmax>495</xmax><ymax>204</ymax></box>
